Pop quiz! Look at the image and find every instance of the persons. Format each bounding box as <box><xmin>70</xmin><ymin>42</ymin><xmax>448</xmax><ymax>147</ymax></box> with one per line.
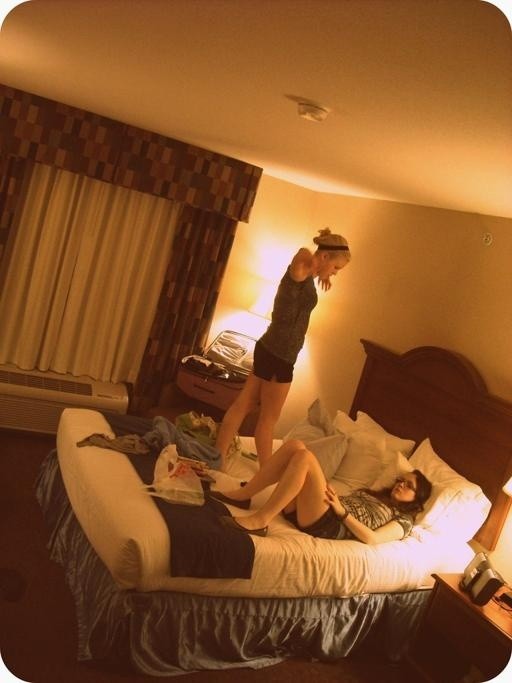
<box><xmin>210</xmin><ymin>437</ymin><xmax>433</xmax><ymax>545</ymax></box>
<box><xmin>212</xmin><ymin>227</ymin><xmax>352</xmax><ymax>478</ymax></box>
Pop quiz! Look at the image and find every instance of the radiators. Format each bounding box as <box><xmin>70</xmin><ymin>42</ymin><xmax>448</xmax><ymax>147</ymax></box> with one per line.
<box><xmin>0</xmin><ymin>366</ymin><xmax>134</xmax><ymax>435</ymax></box>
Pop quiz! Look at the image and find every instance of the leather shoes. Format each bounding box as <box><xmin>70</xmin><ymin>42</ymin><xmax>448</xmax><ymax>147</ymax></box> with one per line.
<box><xmin>217</xmin><ymin>513</ymin><xmax>268</xmax><ymax>537</ymax></box>
<box><xmin>209</xmin><ymin>489</ymin><xmax>252</xmax><ymax>510</ymax></box>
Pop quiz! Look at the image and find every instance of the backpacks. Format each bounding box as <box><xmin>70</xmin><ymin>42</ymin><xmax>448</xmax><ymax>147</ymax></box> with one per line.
<box><xmin>174</xmin><ymin>408</ymin><xmax>241</xmax><ymax>457</ymax></box>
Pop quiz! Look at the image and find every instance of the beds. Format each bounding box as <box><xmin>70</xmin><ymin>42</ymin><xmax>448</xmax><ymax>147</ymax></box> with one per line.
<box><xmin>34</xmin><ymin>338</ymin><xmax>512</xmax><ymax>675</ymax></box>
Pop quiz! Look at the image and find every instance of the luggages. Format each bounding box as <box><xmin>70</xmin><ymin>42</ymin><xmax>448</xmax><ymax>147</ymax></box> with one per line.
<box><xmin>173</xmin><ymin>327</ymin><xmax>259</xmax><ymax>412</ymax></box>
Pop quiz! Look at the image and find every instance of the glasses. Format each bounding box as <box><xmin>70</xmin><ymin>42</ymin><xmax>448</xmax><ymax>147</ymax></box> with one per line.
<box><xmin>396</xmin><ymin>474</ymin><xmax>417</xmax><ymax>494</ymax></box>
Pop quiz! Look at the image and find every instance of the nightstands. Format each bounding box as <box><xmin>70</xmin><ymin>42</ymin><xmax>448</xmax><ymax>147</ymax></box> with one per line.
<box><xmin>170</xmin><ymin>366</ymin><xmax>261</xmax><ymax>436</ymax></box>
<box><xmin>405</xmin><ymin>574</ymin><xmax>512</xmax><ymax>682</ymax></box>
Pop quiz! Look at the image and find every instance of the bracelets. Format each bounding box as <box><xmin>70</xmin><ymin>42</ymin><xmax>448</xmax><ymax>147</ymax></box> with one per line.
<box><xmin>336</xmin><ymin>506</ymin><xmax>349</xmax><ymax>522</ymax></box>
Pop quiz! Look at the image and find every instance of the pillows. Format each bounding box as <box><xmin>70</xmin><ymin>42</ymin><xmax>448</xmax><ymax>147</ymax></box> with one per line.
<box><xmin>283</xmin><ymin>399</ymin><xmax>493</xmax><ymax>546</ymax></box>
